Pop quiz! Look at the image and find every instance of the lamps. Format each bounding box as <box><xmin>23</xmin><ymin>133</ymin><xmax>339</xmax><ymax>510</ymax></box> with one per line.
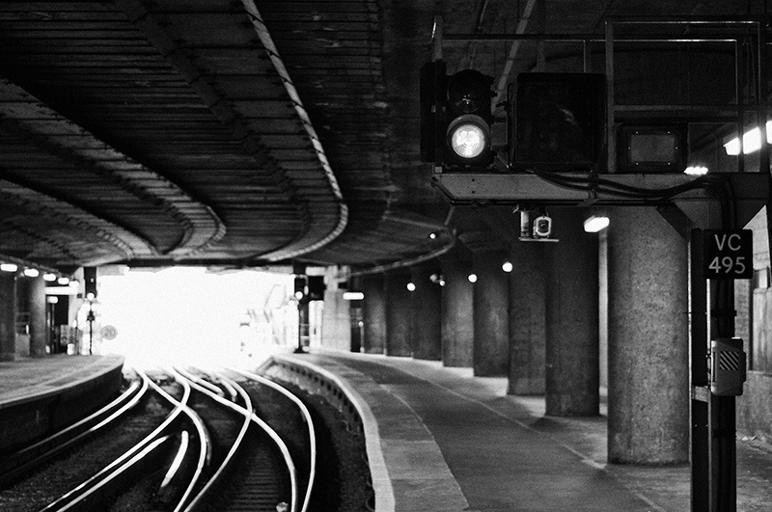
<box><xmin>446</xmin><ymin>69</ymin><xmax>494</xmax><ymax>162</ymax></box>
<box><xmin>582</xmin><ymin>206</ymin><xmax>610</xmax><ymax>233</ymax></box>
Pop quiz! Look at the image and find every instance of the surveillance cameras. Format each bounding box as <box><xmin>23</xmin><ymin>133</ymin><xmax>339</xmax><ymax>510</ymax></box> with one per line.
<box><xmin>532</xmin><ymin>216</ymin><xmax>552</xmax><ymax>239</ymax></box>
